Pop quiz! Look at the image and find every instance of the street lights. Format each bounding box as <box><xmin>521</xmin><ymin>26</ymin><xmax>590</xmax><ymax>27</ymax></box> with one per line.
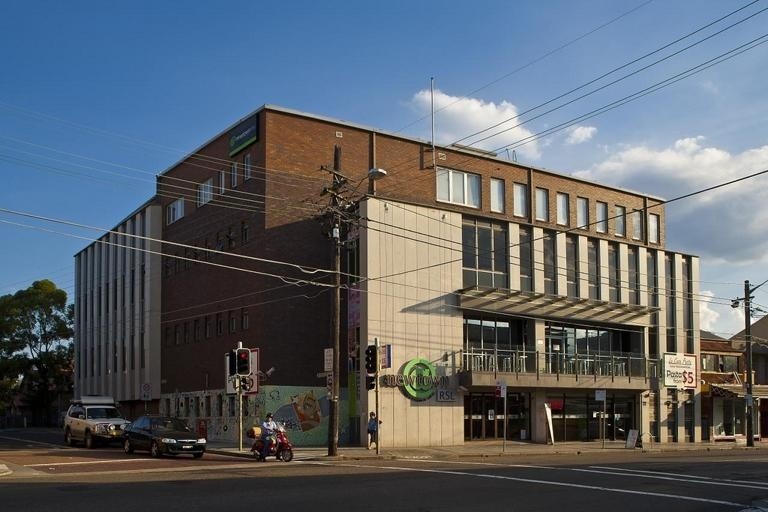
<box><xmin>725</xmin><ymin>293</ymin><xmax>754</xmax><ymax>448</ymax></box>
<box><xmin>321</xmin><ymin>163</ymin><xmax>390</xmax><ymax>457</ymax></box>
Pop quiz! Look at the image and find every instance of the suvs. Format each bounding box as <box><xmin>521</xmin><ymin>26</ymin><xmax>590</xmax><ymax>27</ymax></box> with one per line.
<box><xmin>60</xmin><ymin>391</ymin><xmax>133</xmax><ymax>451</ymax></box>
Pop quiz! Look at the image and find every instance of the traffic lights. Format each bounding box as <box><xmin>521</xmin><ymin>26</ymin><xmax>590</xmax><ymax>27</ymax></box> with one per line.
<box><xmin>363</xmin><ymin>344</ymin><xmax>376</xmax><ymax>373</ymax></box>
<box><xmin>234</xmin><ymin>347</ymin><xmax>251</xmax><ymax>377</ymax></box>
<box><xmin>366</xmin><ymin>376</ymin><xmax>375</xmax><ymax>390</ymax></box>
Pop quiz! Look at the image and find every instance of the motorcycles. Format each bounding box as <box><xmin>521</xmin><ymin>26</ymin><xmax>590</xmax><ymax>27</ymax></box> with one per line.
<box><xmin>245</xmin><ymin>424</ymin><xmax>294</xmax><ymax>463</ymax></box>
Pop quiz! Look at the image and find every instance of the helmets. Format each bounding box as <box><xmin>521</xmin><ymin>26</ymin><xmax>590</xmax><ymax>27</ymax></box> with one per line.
<box><xmin>267</xmin><ymin>412</ymin><xmax>273</xmax><ymax>418</ymax></box>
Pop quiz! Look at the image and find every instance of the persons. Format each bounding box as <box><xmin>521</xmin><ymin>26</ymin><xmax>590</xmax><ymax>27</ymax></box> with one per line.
<box><xmin>258</xmin><ymin>412</ymin><xmax>282</xmax><ymax>462</ymax></box>
<box><xmin>367</xmin><ymin>411</ymin><xmax>383</xmax><ymax>449</ymax></box>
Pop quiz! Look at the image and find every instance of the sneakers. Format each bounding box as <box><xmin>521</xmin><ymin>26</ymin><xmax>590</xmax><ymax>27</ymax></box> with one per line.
<box><xmin>276</xmin><ymin>456</ymin><xmax>282</xmax><ymax>460</ymax></box>
<box><xmin>262</xmin><ymin>457</ymin><xmax>266</xmax><ymax>462</ymax></box>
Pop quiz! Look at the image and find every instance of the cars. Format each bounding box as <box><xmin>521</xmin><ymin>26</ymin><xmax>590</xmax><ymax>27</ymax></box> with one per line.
<box><xmin>118</xmin><ymin>412</ymin><xmax>207</xmax><ymax>460</ymax></box>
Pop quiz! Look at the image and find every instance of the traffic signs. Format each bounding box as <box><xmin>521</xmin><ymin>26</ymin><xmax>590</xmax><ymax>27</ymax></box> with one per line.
<box><xmin>326</xmin><ymin>372</ymin><xmax>333</xmax><ymax>401</ymax></box>
